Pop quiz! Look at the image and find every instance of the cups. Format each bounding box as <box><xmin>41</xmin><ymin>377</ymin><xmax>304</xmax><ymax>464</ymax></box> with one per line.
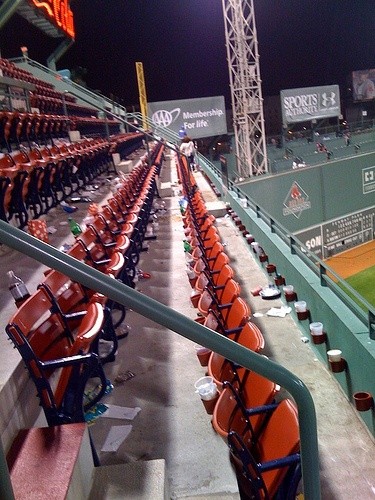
<box><xmin>294</xmin><ymin>301</ymin><xmax>306</xmax><ymax>312</ymax></box>
<box><xmin>283</xmin><ymin>285</ymin><xmax>294</xmax><ymax>294</ymax></box>
<box><xmin>310</xmin><ymin>322</ymin><xmax>324</xmax><ymax>335</ymax></box>
<box><xmin>193</xmin><ymin>344</ymin><xmax>209</xmax><ymax>353</ymax></box>
<box><xmin>194</xmin><ymin>375</ymin><xmax>218</xmax><ymax>400</ymax></box>
<box><xmin>251</xmin><ymin>242</ymin><xmax>259</xmax><ymax>251</ymax></box>
<box><xmin>327</xmin><ymin>349</ymin><xmax>342</xmax><ymax>361</ymax></box>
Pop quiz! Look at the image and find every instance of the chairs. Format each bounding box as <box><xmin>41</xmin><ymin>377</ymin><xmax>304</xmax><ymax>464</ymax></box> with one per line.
<box><xmin>0</xmin><ymin>60</ymin><xmax>168</xmax><ymax>467</ymax></box>
<box><xmin>175</xmin><ymin>155</ymin><xmax>303</xmax><ymax>500</ymax></box>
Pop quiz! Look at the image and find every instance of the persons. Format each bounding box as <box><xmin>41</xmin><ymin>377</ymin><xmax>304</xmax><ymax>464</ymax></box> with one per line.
<box><xmin>178</xmin><ymin>125</ymin><xmax>185</xmax><ymax>140</ymax></box>
<box><xmin>341</xmin><ymin>132</ymin><xmax>352</xmax><ymax>152</ymax></box>
<box><xmin>179</xmin><ymin>136</ymin><xmax>197</xmax><ymax>172</ymax></box>
<box><xmin>356</xmin><ymin>71</ymin><xmax>375</xmax><ymax>101</ymax></box>
<box><xmin>306</xmin><ymin>138</ymin><xmax>335</xmax><ymax>158</ymax></box>
<box><xmin>284</xmin><ymin>144</ymin><xmax>307</xmax><ymax>166</ymax></box>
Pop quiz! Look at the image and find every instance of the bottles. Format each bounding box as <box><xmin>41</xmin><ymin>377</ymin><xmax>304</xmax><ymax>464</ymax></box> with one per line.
<box><xmin>32</xmin><ymin>142</ymin><xmax>39</xmax><ymax>149</ymax></box>
<box><xmin>68</xmin><ymin>217</ymin><xmax>82</xmax><ymax>236</ymax></box>
<box><xmin>6</xmin><ymin>270</ymin><xmax>30</xmax><ymax>300</ymax></box>
<box><xmin>19</xmin><ymin>143</ymin><xmax>27</xmax><ymax>151</ymax></box>
<box><xmin>183</xmin><ymin>239</ymin><xmax>192</xmax><ymax>252</ymax></box>
